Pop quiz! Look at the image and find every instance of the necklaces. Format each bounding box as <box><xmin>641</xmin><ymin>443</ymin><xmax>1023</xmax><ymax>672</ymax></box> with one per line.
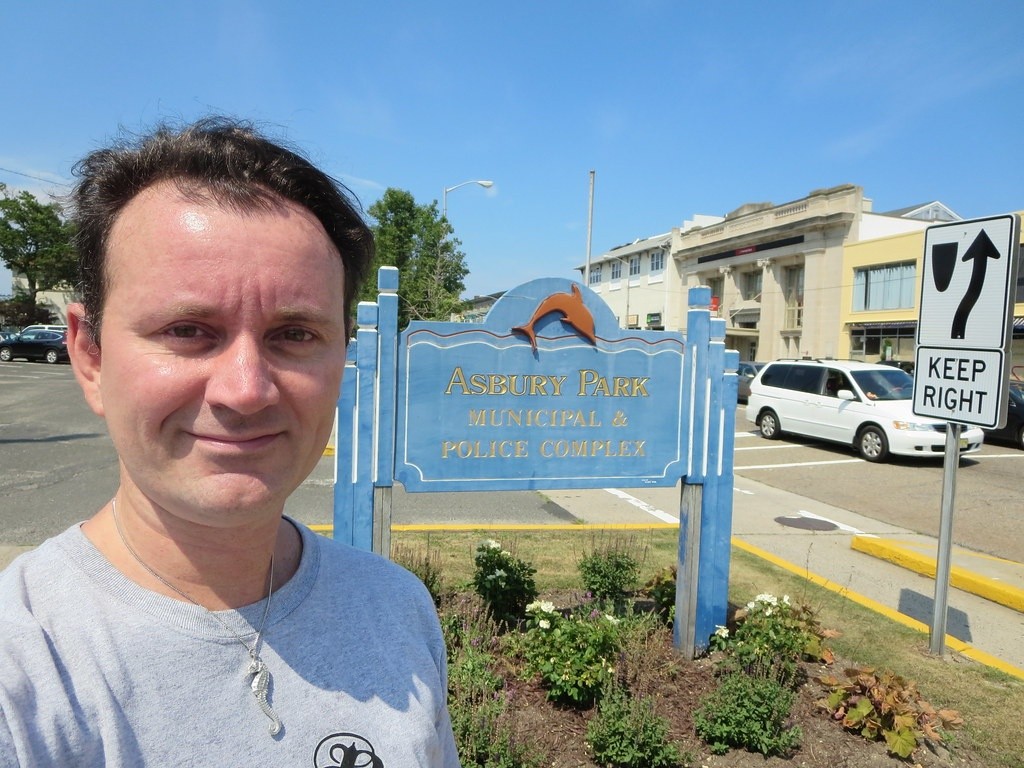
<box><xmin>111</xmin><ymin>494</ymin><xmax>281</xmax><ymax>735</ymax></box>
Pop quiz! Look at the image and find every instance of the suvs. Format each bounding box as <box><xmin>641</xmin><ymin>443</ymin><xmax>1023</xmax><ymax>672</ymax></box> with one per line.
<box><xmin>746</xmin><ymin>356</ymin><xmax>984</xmax><ymax>462</ymax></box>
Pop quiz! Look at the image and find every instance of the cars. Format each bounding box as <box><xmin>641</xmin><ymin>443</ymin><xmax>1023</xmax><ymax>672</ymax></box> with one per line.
<box><xmin>0</xmin><ymin>328</ymin><xmax>71</xmax><ymax>364</ymax></box>
<box><xmin>738</xmin><ymin>359</ymin><xmax>766</xmax><ymax>404</ymax></box>
<box><xmin>876</xmin><ymin>361</ymin><xmax>916</xmax><ymax>378</ymax></box>
<box><xmin>981</xmin><ymin>380</ymin><xmax>1024</xmax><ymax>450</ymax></box>
<box><xmin>0</xmin><ymin>331</ymin><xmax>13</xmax><ymax>344</ymax></box>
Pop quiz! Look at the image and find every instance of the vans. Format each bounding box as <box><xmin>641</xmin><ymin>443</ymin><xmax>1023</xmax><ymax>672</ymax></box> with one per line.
<box><xmin>9</xmin><ymin>325</ymin><xmax>69</xmax><ymax>340</ymax></box>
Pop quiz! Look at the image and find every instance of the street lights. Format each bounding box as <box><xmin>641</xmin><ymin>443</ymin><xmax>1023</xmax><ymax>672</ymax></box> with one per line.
<box><xmin>438</xmin><ymin>179</ymin><xmax>493</xmax><ymax>259</ymax></box>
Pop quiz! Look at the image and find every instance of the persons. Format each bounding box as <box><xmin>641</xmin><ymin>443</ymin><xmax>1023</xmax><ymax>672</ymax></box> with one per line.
<box><xmin>0</xmin><ymin>116</ymin><xmax>460</xmax><ymax>768</ymax></box>
